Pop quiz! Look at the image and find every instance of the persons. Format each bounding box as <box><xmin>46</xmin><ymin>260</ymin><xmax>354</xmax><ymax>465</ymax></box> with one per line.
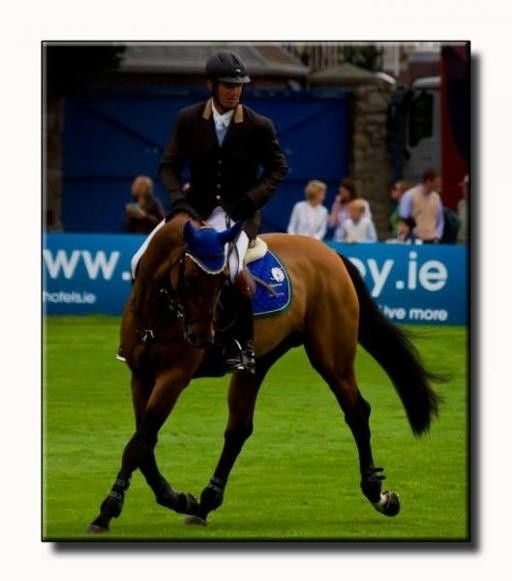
<box><xmin>328</xmin><ymin>178</ymin><xmax>372</xmax><ymax>241</ymax></box>
<box><xmin>116</xmin><ymin>51</ymin><xmax>289</xmax><ymax>377</ymax></box>
<box><xmin>287</xmin><ymin>180</ymin><xmax>328</xmax><ymax>240</ymax></box>
<box><xmin>384</xmin><ymin>172</ymin><xmax>469</xmax><ymax>245</ymax></box>
<box><xmin>125</xmin><ymin>177</ymin><xmax>165</xmax><ymax>233</ymax></box>
<box><xmin>335</xmin><ymin>200</ymin><xmax>378</xmax><ymax>244</ymax></box>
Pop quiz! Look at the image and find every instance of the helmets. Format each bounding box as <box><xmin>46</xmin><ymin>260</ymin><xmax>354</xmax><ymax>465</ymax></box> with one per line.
<box><xmin>205</xmin><ymin>50</ymin><xmax>251</xmax><ymax>85</ymax></box>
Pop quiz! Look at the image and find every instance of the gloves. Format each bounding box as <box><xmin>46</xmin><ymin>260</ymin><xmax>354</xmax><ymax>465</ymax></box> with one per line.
<box><xmin>169</xmin><ymin>197</ymin><xmax>202</xmax><ymax>220</ymax></box>
<box><xmin>226</xmin><ymin>194</ymin><xmax>250</xmax><ymax>221</ymax></box>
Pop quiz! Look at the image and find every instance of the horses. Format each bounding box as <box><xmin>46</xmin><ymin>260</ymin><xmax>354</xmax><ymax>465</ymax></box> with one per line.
<box><xmin>85</xmin><ymin>212</ymin><xmax>445</xmax><ymax>534</ymax></box>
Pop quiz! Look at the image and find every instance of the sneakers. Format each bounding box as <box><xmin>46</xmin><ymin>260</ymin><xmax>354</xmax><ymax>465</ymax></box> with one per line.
<box><xmin>225</xmin><ymin>349</ymin><xmax>256</xmax><ymax>376</ymax></box>
